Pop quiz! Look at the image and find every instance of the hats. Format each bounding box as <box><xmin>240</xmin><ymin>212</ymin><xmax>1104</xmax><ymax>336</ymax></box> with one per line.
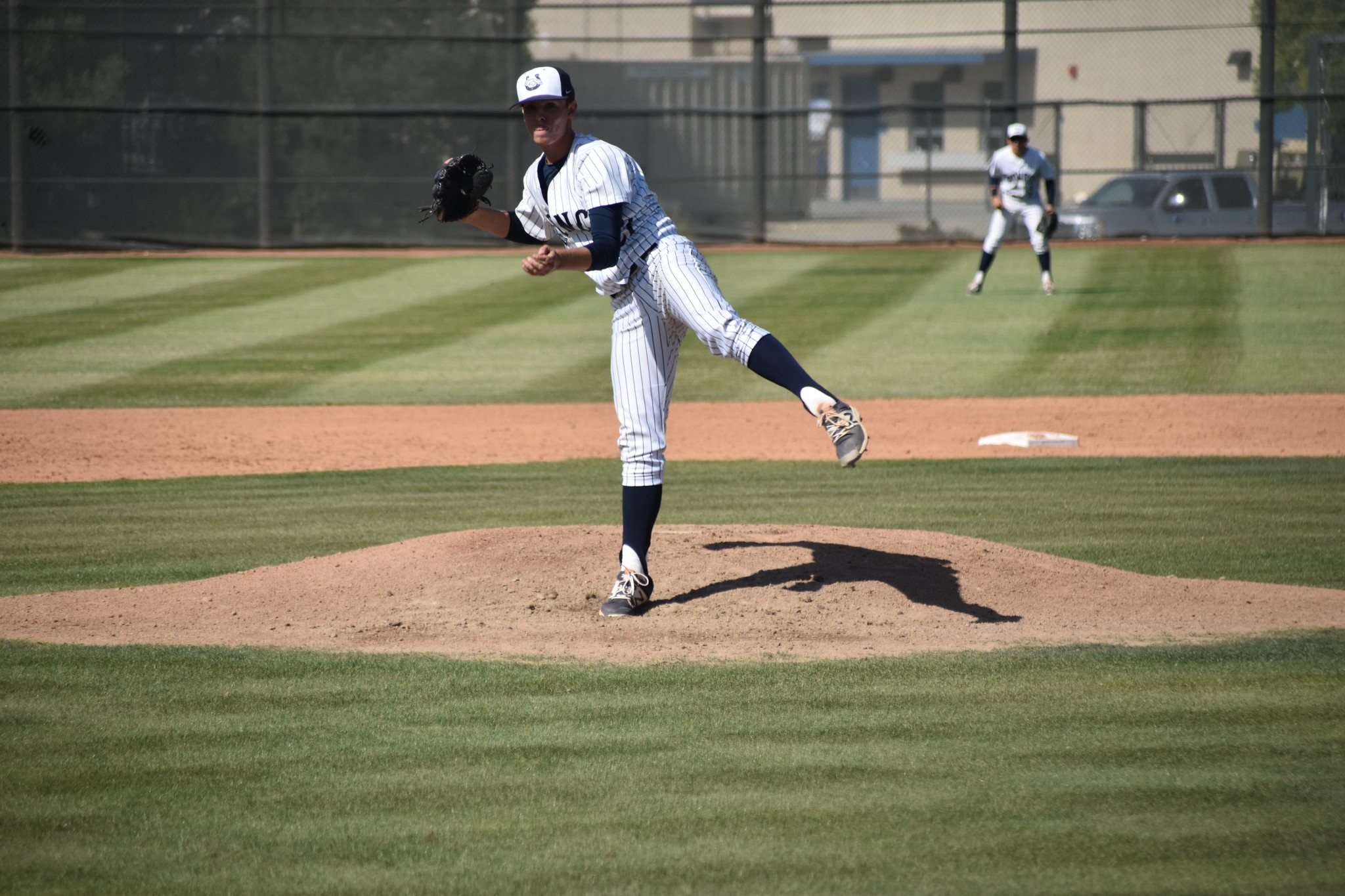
<box><xmin>1007</xmin><ymin>122</ymin><xmax>1028</xmax><ymax>138</ymax></box>
<box><xmin>509</xmin><ymin>66</ymin><xmax>574</xmax><ymax>111</ymax></box>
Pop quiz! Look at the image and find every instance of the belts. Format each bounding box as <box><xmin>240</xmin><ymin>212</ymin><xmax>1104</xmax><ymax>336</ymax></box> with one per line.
<box><xmin>610</xmin><ymin>243</ymin><xmax>658</xmax><ymax>299</ymax></box>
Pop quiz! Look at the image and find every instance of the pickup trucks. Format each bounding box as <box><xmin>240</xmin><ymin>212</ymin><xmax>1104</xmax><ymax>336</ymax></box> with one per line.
<box><xmin>1052</xmin><ymin>170</ymin><xmax>1345</xmax><ymax>242</ymax></box>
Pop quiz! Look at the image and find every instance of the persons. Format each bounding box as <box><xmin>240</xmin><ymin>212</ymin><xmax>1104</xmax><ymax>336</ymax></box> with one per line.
<box><xmin>441</xmin><ymin>66</ymin><xmax>869</xmax><ymax>616</ymax></box>
<box><xmin>969</xmin><ymin>122</ymin><xmax>1058</xmax><ymax>295</ymax></box>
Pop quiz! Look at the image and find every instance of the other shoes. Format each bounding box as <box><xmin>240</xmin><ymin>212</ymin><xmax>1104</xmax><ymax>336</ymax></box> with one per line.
<box><xmin>1043</xmin><ymin>282</ymin><xmax>1054</xmax><ymax>293</ymax></box>
<box><xmin>969</xmin><ymin>283</ymin><xmax>982</xmax><ymax>292</ymax></box>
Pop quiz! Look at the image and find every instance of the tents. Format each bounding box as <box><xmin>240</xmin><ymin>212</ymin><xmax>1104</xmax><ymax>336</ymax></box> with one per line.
<box><xmin>1254</xmin><ymin>104</ymin><xmax>1321</xmax><ymax>197</ymax></box>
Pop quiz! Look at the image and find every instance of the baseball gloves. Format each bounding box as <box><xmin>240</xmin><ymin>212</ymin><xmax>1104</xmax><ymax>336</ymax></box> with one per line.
<box><xmin>1036</xmin><ymin>209</ymin><xmax>1060</xmax><ymax>235</ymax></box>
<box><xmin>431</xmin><ymin>152</ymin><xmax>494</xmax><ymax>224</ymax></box>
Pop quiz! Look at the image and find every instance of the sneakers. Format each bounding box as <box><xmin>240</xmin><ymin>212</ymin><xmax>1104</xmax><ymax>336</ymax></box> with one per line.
<box><xmin>815</xmin><ymin>399</ymin><xmax>869</xmax><ymax>469</ymax></box>
<box><xmin>598</xmin><ymin>563</ymin><xmax>654</xmax><ymax>617</ymax></box>
